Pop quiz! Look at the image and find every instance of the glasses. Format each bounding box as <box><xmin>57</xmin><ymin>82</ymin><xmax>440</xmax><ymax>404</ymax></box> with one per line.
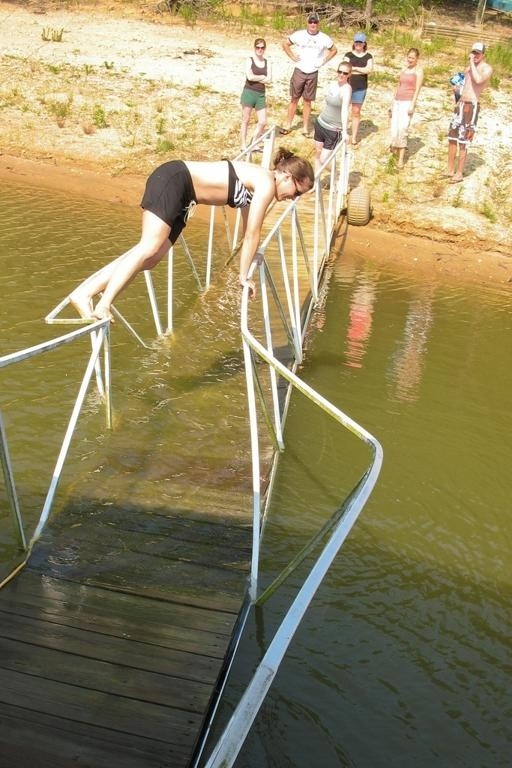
<box><xmin>337</xmin><ymin>71</ymin><xmax>349</xmax><ymax>75</ymax></box>
<box><xmin>293</xmin><ymin>177</ymin><xmax>303</xmax><ymax>197</ymax></box>
<box><xmin>309</xmin><ymin>21</ymin><xmax>317</xmax><ymax>24</ymax></box>
<box><xmin>255</xmin><ymin>47</ymin><xmax>265</xmax><ymax>49</ymax></box>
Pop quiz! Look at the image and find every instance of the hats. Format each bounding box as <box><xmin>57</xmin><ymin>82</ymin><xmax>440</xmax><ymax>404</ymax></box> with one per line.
<box><xmin>469</xmin><ymin>42</ymin><xmax>486</xmax><ymax>54</ymax></box>
<box><xmin>353</xmin><ymin>32</ymin><xmax>367</xmax><ymax>43</ymax></box>
<box><xmin>307</xmin><ymin>12</ymin><xmax>320</xmax><ymax>21</ymax></box>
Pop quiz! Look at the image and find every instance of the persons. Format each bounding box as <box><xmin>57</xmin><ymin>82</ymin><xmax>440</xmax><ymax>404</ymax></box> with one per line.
<box><xmin>386</xmin><ymin>48</ymin><xmax>424</xmax><ymax>169</ymax></box>
<box><xmin>238</xmin><ymin>37</ymin><xmax>274</xmax><ymax>156</ymax></box>
<box><xmin>310</xmin><ymin>61</ymin><xmax>354</xmax><ymax>186</ymax></box>
<box><xmin>439</xmin><ymin>43</ymin><xmax>493</xmax><ymax>183</ymax></box>
<box><xmin>66</xmin><ymin>146</ymin><xmax>315</xmax><ymax>327</ymax></box>
<box><xmin>340</xmin><ymin>33</ymin><xmax>375</xmax><ymax>145</ymax></box>
<box><xmin>278</xmin><ymin>14</ymin><xmax>338</xmax><ymax>138</ymax></box>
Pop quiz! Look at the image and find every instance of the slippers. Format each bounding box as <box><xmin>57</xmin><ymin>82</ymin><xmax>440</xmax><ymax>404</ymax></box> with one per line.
<box><xmin>279</xmin><ymin>128</ymin><xmax>291</xmax><ymax>135</ymax></box>
<box><xmin>301</xmin><ymin>132</ymin><xmax>313</xmax><ymax>139</ymax></box>
<box><xmin>251</xmin><ymin>148</ymin><xmax>263</xmax><ymax>153</ymax></box>
<box><xmin>437</xmin><ymin>174</ymin><xmax>464</xmax><ymax>184</ymax></box>
